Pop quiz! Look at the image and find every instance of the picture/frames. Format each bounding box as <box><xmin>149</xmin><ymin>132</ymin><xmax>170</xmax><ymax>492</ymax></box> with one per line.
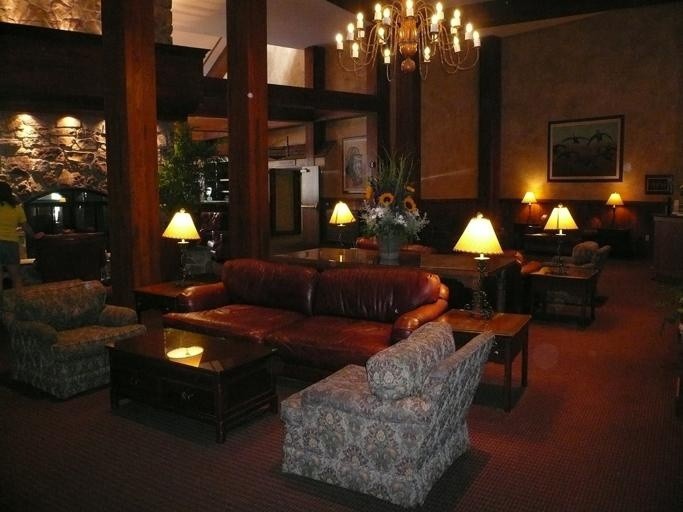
<box><xmin>644</xmin><ymin>175</ymin><xmax>674</xmax><ymax>195</ymax></box>
<box><xmin>546</xmin><ymin>114</ymin><xmax>624</xmax><ymax>183</ymax></box>
<box><xmin>342</xmin><ymin>135</ymin><xmax>367</xmax><ymax>194</ymax></box>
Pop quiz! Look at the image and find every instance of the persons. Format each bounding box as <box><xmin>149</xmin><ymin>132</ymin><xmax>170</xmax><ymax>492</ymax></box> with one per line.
<box><xmin>0</xmin><ymin>183</ymin><xmax>44</xmax><ymax>289</ymax></box>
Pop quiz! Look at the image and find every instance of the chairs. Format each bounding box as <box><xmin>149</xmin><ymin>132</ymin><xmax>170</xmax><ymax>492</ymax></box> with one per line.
<box><xmin>540</xmin><ymin>240</ymin><xmax>612</xmax><ymax>305</ymax></box>
<box><xmin>0</xmin><ymin>279</ymin><xmax>148</xmax><ymax>401</ymax></box>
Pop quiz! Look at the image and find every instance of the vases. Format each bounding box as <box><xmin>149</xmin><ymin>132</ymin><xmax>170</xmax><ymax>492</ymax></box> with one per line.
<box><xmin>375</xmin><ymin>223</ymin><xmax>404</xmax><ymax>266</ymax></box>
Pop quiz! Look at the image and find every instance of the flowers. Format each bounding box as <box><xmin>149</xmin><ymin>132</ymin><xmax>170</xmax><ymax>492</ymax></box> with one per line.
<box><xmin>359</xmin><ymin>145</ymin><xmax>430</xmax><ymax>242</ymax></box>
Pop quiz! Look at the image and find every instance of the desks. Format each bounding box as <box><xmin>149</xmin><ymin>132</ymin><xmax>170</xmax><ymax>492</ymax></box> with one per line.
<box><xmin>104</xmin><ymin>327</ymin><xmax>280</xmax><ymax>444</ymax></box>
<box><xmin>275</xmin><ymin>246</ymin><xmax>518</xmax><ymax>313</ymax></box>
<box><xmin>647</xmin><ymin>212</ymin><xmax>683</xmax><ymax>282</ymax></box>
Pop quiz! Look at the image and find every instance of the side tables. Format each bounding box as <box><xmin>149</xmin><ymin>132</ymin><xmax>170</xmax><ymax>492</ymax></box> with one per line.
<box><xmin>526</xmin><ymin>266</ymin><xmax>599</xmax><ymax>327</ymax></box>
<box><xmin>435</xmin><ymin>307</ymin><xmax>533</xmax><ymax>414</ymax></box>
<box><xmin>131</xmin><ymin>279</ymin><xmax>214</xmax><ymax>324</ymax></box>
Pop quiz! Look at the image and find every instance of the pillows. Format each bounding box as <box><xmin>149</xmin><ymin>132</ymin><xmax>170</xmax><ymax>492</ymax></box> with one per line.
<box><xmin>365</xmin><ymin>321</ymin><xmax>456</xmax><ymax>400</ymax></box>
<box><xmin>572</xmin><ymin>240</ymin><xmax>599</xmax><ymax>267</ymax></box>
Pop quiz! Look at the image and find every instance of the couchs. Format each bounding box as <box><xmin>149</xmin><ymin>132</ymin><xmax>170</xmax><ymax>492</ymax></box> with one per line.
<box><xmin>161</xmin><ymin>258</ymin><xmax>451</xmax><ymax>383</ymax></box>
<box><xmin>280</xmin><ymin>320</ymin><xmax>496</xmax><ymax>509</ymax></box>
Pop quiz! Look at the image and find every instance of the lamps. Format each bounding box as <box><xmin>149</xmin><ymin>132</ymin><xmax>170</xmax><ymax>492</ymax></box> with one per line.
<box><xmin>333</xmin><ymin>0</ymin><xmax>483</xmax><ymax>83</ymax></box>
<box><xmin>161</xmin><ymin>207</ymin><xmax>202</xmax><ymax>288</ymax></box>
<box><xmin>521</xmin><ymin>192</ymin><xmax>538</xmax><ymax>223</ymax></box>
<box><xmin>606</xmin><ymin>192</ymin><xmax>625</xmax><ymax>227</ymax></box>
<box><xmin>542</xmin><ymin>202</ymin><xmax>580</xmax><ymax>275</ymax></box>
<box><xmin>453</xmin><ymin>212</ymin><xmax>505</xmax><ymax>320</ymax></box>
<box><xmin>328</xmin><ymin>200</ymin><xmax>356</xmax><ymax>249</ymax></box>
